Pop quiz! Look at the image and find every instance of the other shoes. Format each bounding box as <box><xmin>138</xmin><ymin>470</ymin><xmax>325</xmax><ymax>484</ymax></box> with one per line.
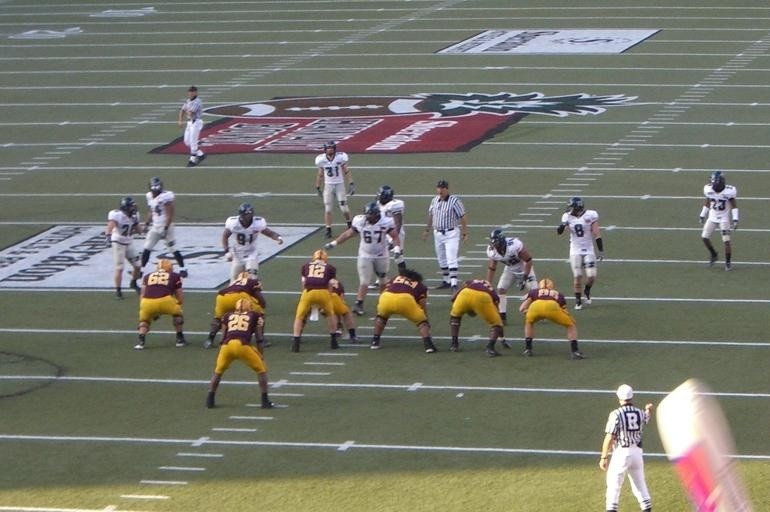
<box><xmin>521</xmin><ymin>349</ymin><xmax>534</xmax><ymax>357</ymax></box>
<box><xmin>134</xmin><ymin>337</ymin><xmax>146</xmax><ymax>349</ymax></box>
<box><xmin>423</xmin><ymin>337</ymin><xmax>435</xmax><ymax>353</ymax></box>
<box><xmin>259</xmin><ymin>339</ymin><xmax>272</xmax><ymax>347</ymax></box>
<box><xmin>448</xmin><ymin>343</ymin><xmax>459</xmax><ymax>352</ymax></box>
<box><xmin>330</xmin><ymin>339</ymin><xmax>339</xmax><ymax>350</ymax></box>
<box><xmin>487</xmin><ymin>341</ymin><xmax>500</xmax><ymax>357</ymax></box>
<box><xmin>571</xmin><ymin>351</ymin><xmax>588</xmax><ymax>359</ymax></box>
<box><xmin>176</xmin><ymin>333</ymin><xmax>186</xmax><ymax>346</ymax></box>
<box><xmin>205</xmin><ymin>338</ymin><xmax>214</xmax><ymax>349</ymax></box>
<box><xmin>291</xmin><ymin>336</ymin><xmax>301</xmax><ymax>353</ymax></box>
<box><xmin>370</xmin><ymin>337</ymin><xmax>380</xmax><ymax>349</ymax></box>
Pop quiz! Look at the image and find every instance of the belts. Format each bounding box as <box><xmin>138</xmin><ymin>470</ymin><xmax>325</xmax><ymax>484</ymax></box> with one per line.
<box><xmin>437</xmin><ymin>228</ymin><xmax>455</xmax><ymax>234</ymax></box>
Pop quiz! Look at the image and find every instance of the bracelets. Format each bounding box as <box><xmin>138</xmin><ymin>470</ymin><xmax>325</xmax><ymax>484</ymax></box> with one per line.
<box><xmin>601</xmin><ymin>456</ymin><xmax>608</xmax><ymax>459</ymax></box>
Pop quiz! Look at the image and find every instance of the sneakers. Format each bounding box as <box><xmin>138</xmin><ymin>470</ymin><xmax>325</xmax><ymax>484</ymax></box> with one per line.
<box><xmin>323</xmin><ymin>228</ymin><xmax>336</xmax><ymax>241</ymax></box>
<box><xmin>185</xmin><ymin>152</ymin><xmax>209</xmax><ymax>167</ymax></box>
<box><xmin>112</xmin><ymin>280</ymin><xmax>143</xmax><ymax>301</ymax></box>
<box><xmin>704</xmin><ymin>253</ymin><xmax>738</xmax><ymax>274</ymax></box>
<box><xmin>352</xmin><ymin>298</ymin><xmax>366</xmax><ymax>315</ymax></box>
<box><xmin>573</xmin><ymin>286</ymin><xmax>594</xmax><ymax>311</ymax></box>
<box><xmin>435</xmin><ymin>267</ymin><xmax>459</xmax><ymax>292</ymax></box>
<box><xmin>260</xmin><ymin>400</ymin><xmax>276</xmax><ymax>408</ymax></box>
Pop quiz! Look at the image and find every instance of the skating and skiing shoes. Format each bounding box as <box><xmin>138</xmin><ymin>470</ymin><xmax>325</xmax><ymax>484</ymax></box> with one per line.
<box><xmin>204</xmin><ymin>389</ymin><xmax>216</xmax><ymax>410</ymax></box>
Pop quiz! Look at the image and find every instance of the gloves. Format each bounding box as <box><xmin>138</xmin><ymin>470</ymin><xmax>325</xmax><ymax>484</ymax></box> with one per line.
<box><xmin>597</xmin><ymin>250</ymin><xmax>607</xmax><ymax>263</ymax></box>
<box><xmin>348</xmin><ymin>181</ymin><xmax>357</xmax><ymax>196</ymax></box>
<box><xmin>391</xmin><ymin>245</ymin><xmax>401</xmax><ymax>259</ymax></box>
<box><xmin>316</xmin><ymin>184</ymin><xmax>325</xmax><ymax>198</ymax></box>
<box><xmin>321</xmin><ymin>240</ymin><xmax>338</xmax><ymax>249</ymax></box>
<box><xmin>501</xmin><ymin>340</ymin><xmax>511</xmax><ymax>349</ymax></box>
<box><xmin>102</xmin><ymin>221</ymin><xmax>171</xmax><ymax>249</ymax></box>
<box><xmin>517</xmin><ymin>274</ymin><xmax>527</xmax><ymax>290</ymax></box>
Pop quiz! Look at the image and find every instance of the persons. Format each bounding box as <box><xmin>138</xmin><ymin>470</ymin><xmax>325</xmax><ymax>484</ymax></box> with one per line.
<box><xmin>519</xmin><ymin>278</ymin><xmax>586</xmax><ymax>360</ymax></box>
<box><xmin>291</xmin><ymin>185</ymin><xmax>409</xmax><ymax>353</ymax></box>
<box><xmin>486</xmin><ymin>229</ymin><xmax>538</xmax><ymax>326</ymax></box>
<box><xmin>370</xmin><ymin>269</ymin><xmax>439</xmax><ymax>354</ymax></box>
<box><xmin>204</xmin><ymin>202</ymin><xmax>284</xmax><ymax>412</ymax></box>
<box><xmin>556</xmin><ymin>197</ymin><xmax>604</xmax><ymax>310</ymax></box>
<box><xmin>599</xmin><ymin>383</ymin><xmax>654</xmax><ymax>512</ymax></box>
<box><xmin>421</xmin><ymin>179</ymin><xmax>470</xmax><ymax>293</ymax></box>
<box><xmin>448</xmin><ymin>278</ymin><xmax>511</xmax><ymax>358</ymax></box>
<box><xmin>103</xmin><ymin>176</ymin><xmax>188</xmax><ymax>348</ymax></box>
<box><xmin>314</xmin><ymin>141</ymin><xmax>356</xmax><ymax>239</ymax></box>
<box><xmin>698</xmin><ymin>171</ymin><xmax>739</xmax><ymax>271</ymax></box>
<box><xmin>177</xmin><ymin>87</ymin><xmax>206</xmax><ymax>167</ymax></box>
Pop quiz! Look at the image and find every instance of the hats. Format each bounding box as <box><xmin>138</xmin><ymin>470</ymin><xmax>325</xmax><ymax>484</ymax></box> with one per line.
<box><xmin>434</xmin><ymin>177</ymin><xmax>450</xmax><ymax>191</ymax></box>
<box><xmin>615</xmin><ymin>383</ymin><xmax>634</xmax><ymax>402</ymax></box>
<box><xmin>187</xmin><ymin>85</ymin><xmax>197</xmax><ymax>94</ymax></box>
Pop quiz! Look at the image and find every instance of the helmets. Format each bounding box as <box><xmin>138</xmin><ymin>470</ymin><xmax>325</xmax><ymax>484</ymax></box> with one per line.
<box><xmin>488</xmin><ymin>226</ymin><xmax>506</xmax><ymax>250</ymax></box>
<box><xmin>230</xmin><ymin>297</ymin><xmax>255</xmax><ymax>312</ymax></box>
<box><xmin>116</xmin><ymin>196</ymin><xmax>138</xmax><ymax>217</ymax></box>
<box><xmin>566</xmin><ymin>194</ymin><xmax>585</xmax><ymax>216</ymax></box>
<box><xmin>712</xmin><ymin>168</ymin><xmax>727</xmax><ymax>192</ymax></box>
<box><xmin>365</xmin><ymin>201</ymin><xmax>382</xmax><ymax>223</ymax></box>
<box><xmin>378</xmin><ymin>183</ymin><xmax>394</xmax><ymax>204</ymax></box>
<box><xmin>147</xmin><ymin>175</ymin><xmax>165</xmax><ymax>194</ymax></box>
<box><xmin>538</xmin><ymin>276</ymin><xmax>555</xmax><ymax>290</ymax></box>
<box><xmin>323</xmin><ymin>139</ymin><xmax>336</xmax><ymax>158</ymax></box>
<box><xmin>156</xmin><ymin>257</ymin><xmax>173</xmax><ymax>271</ymax></box>
<box><xmin>236</xmin><ymin>201</ymin><xmax>254</xmax><ymax>219</ymax></box>
<box><xmin>311</xmin><ymin>248</ymin><xmax>329</xmax><ymax>262</ymax></box>
